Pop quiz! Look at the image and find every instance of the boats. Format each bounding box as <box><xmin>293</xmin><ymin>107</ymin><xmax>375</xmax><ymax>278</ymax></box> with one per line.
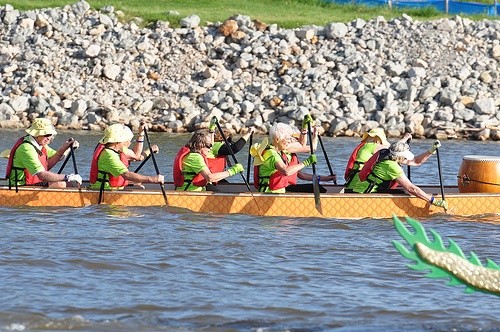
<box><xmin>391</xmin><ymin>212</ymin><xmax>500</xmax><ymax>297</ymax></box>
<box><xmin>0</xmin><ymin>177</ymin><xmax>500</xmax><ymax>220</ymax></box>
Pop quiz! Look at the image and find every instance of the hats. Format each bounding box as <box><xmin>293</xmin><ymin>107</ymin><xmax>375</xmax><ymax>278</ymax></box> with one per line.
<box><xmin>98</xmin><ymin>124</ymin><xmax>131</xmax><ymax>144</ymax></box>
<box><xmin>250</xmin><ymin>137</ymin><xmax>268</xmax><ymax>166</ymax></box>
<box><xmin>25</xmin><ymin>118</ymin><xmax>57</xmax><ymax>137</ymax></box>
<box><xmin>363</xmin><ymin>127</ymin><xmax>391</xmax><ymax>147</ymax></box>
<box><xmin>389</xmin><ymin>147</ymin><xmax>414</xmax><ymax>160</ymax></box>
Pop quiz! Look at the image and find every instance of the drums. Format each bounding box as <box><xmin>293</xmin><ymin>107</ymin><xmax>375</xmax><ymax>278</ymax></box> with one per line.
<box><xmin>457</xmin><ymin>155</ymin><xmax>500</xmax><ymax>194</ymax></box>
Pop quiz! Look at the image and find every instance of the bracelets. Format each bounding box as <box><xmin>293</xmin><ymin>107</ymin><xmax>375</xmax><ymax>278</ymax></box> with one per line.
<box><xmin>209</xmin><ymin>130</ymin><xmax>215</xmax><ymax>134</ymax></box>
<box><xmin>301</xmin><ymin>131</ymin><xmax>307</xmax><ymax>135</ymax></box>
<box><xmin>317</xmin><ymin>175</ymin><xmax>320</xmax><ymax>183</ymax></box>
<box><xmin>137</xmin><ymin>139</ymin><xmax>144</xmax><ymax>142</ymax></box>
<box><xmin>64</xmin><ymin>174</ymin><xmax>68</xmax><ymax>181</ymax></box>
<box><xmin>430</xmin><ymin>197</ymin><xmax>435</xmax><ymax>204</ymax></box>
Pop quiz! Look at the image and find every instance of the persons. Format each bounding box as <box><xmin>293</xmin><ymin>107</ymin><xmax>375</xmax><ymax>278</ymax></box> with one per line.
<box><xmin>182</xmin><ymin>114</ymin><xmax>254</xmax><ymax>186</ymax></box>
<box><xmin>174</xmin><ymin>117</ymin><xmax>244</xmax><ymax>191</ymax></box>
<box><xmin>120</xmin><ymin>124</ymin><xmax>159</xmax><ymax>172</ymax></box>
<box><xmin>345</xmin><ymin>141</ymin><xmax>446</xmax><ymax>210</ymax></box>
<box><xmin>91</xmin><ymin>122</ymin><xmax>167</xmax><ymax>189</ymax></box>
<box><xmin>253</xmin><ymin>114</ymin><xmax>316</xmax><ymax>192</ymax></box>
<box><xmin>346</xmin><ymin>120</ymin><xmax>412</xmax><ymax>180</ymax></box>
<box><xmin>45</xmin><ymin>124</ymin><xmax>79</xmax><ymax>171</ymax></box>
<box><xmin>276</xmin><ymin>116</ymin><xmax>338</xmax><ymax>182</ymax></box>
<box><xmin>5</xmin><ymin>118</ymin><xmax>82</xmax><ymax>188</ymax></box>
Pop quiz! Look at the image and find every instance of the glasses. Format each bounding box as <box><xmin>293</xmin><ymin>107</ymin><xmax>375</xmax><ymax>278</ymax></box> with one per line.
<box><xmin>206</xmin><ymin>144</ymin><xmax>213</xmax><ymax>149</ymax></box>
<box><xmin>294</xmin><ymin>137</ymin><xmax>299</xmax><ymax>140</ymax></box>
<box><xmin>44</xmin><ymin>134</ymin><xmax>52</xmax><ymax>137</ymax></box>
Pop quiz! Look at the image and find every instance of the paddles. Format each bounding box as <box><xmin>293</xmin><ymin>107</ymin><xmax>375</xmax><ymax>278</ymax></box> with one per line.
<box><xmin>246</xmin><ymin>131</ymin><xmax>254</xmax><ymax>184</ymax></box>
<box><xmin>133</xmin><ymin>150</ymin><xmax>157</xmax><ymax>174</ymax></box>
<box><xmin>406</xmin><ymin>137</ymin><xmax>412</xmax><ymax>182</ymax></box>
<box><xmin>317</xmin><ymin>134</ymin><xmax>338</xmax><ymax>185</ymax></box>
<box><xmin>434</xmin><ymin>142</ymin><xmax>447</xmax><ymax>215</ymax></box>
<box><xmin>304</xmin><ymin>114</ymin><xmax>323</xmax><ymax>216</ymax></box>
<box><xmin>140</xmin><ymin>122</ymin><xmax>171</xmax><ymax>206</ymax></box>
<box><xmin>68</xmin><ymin>138</ymin><xmax>86</xmax><ymax>207</ymax></box>
<box><xmin>57</xmin><ymin>146</ymin><xmax>77</xmax><ymax>174</ymax></box>
<box><xmin>213</xmin><ymin>115</ymin><xmax>261</xmax><ymax>212</ymax></box>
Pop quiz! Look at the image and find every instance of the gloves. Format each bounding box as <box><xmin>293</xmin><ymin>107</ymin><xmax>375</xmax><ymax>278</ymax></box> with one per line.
<box><xmin>302</xmin><ymin>115</ymin><xmax>312</xmax><ymax>128</ymax></box>
<box><xmin>429</xmin><ymin>140</ymin><xmax>441</xmax><ymax>154</ymax></box>
<box><xmin>228</xmin><ymin>164</ymin><xmax>244</xmax><ymax>176</ymax></box>
<box><xmin>67</xmin><ymin>174</ymin><xmax>82</xmax><ymax>185</ymax></box>
<box><xmin>303</xmin><ymin>154</ymin><xmax>317</xmax><ymax>167</ymax></box>
<box><xmin>432</xmin><ymin>198</ymin><xmax>448</xmax><ymax>209</ymax></box>
<box><xmin>209</xmin><ymin>116</ymin><xmax>218</xmax><ymax>130</ymax></box>
